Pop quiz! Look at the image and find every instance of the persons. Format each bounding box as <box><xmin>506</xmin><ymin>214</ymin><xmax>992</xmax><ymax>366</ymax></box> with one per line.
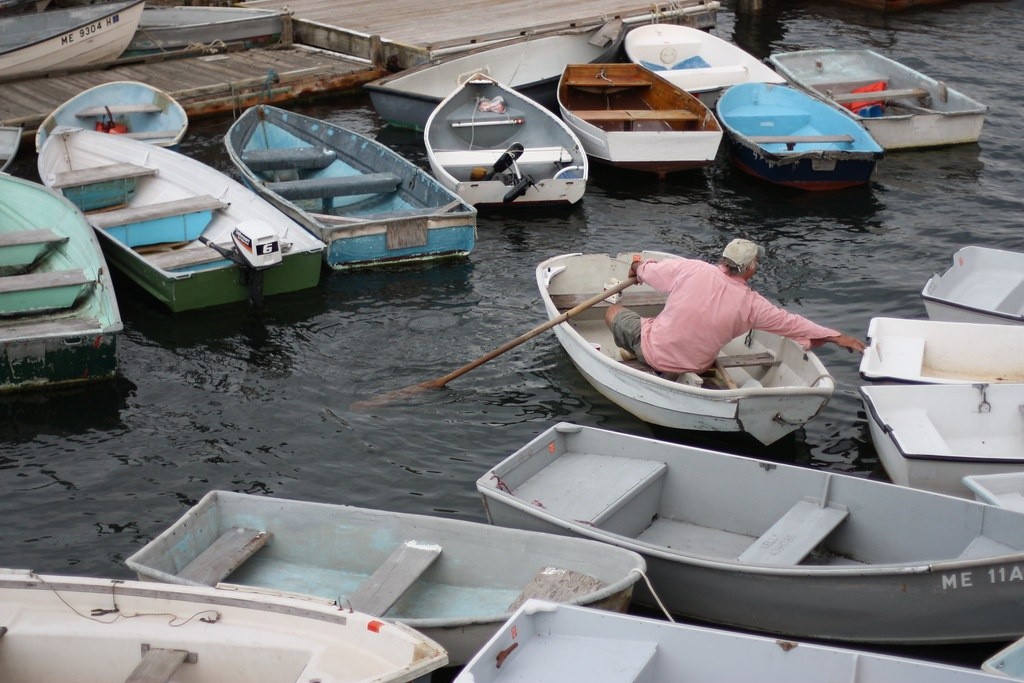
<box><xmin>605</xmin><ymin>237</ymin><xmax>868</xmax><ymax>375</ymax></box>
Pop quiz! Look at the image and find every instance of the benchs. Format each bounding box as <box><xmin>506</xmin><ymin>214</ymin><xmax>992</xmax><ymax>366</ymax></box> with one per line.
<box><xmin>116</xmin><ymin>128</ymin><xmax>176</xmax><ymax>140</ymax></box>
<box><xmin>51</xmin><ymin>162</ymin><xmax>158</xmax><ymax>188</ymax></box>
<box><xmin>135</xmin><ymin>242</ymin><xmax>290</xmax><ymax>268</ymax></box>
<box><xmin>260</xmin><ymin>174</ymin><xmax>401</xmax><ymax>199</ymax></box>
<box><xmin>177</xmin><ymin>527</ymin><xmax>269</xmax><ymax>588</ymax></box>
<box><xmin>742</xmin><ymin>499</ymin><xmax>848</xmax><ymax>567</ymax></box>
<box><xmin>85</xmin><ymin>193</ymin><xmax>228</xmax><ymax>230</ymax></box>
<box><xmin>747</xmin><ymin>135</ymin><xmax>855</xmax><ymax>145</ymax></box>
<box><xmin>0</xmin><ymin>231</ymin><xmax>66</xmax><ymax>248</ymax></box>
<box><xmin>1</xmin><ymin>268</ymin><xmax>91</xmax><ymax>293</ymax></box>
<box><xmin>240</xmin><ymin>146</ymin><xmax>338</xmax><ymax>170</ymax></box>
<box><xmin>654</xmin><ymin>65</ymin><xmax>746</xmax><ymax>89</ymax></box>
<box><xmin>77</xmin><ymin>104</ymin><xmax>161</xmax><ymax>119</ymax></box>
<box><xmin>433</xmin><ymin>145</ymin><xmax>570</xmax><ymax>177</ymax></box>
<box><xmin>567</xmin><ymin>110</ymin><xmax>692</xmax><ymax>127</ymax></box>
<box><xmin>346</xmin><ymin>538</ymin><xmax>441</xmax><ymax>618</ymax></box>
<box><xmin>125</xmin><ymin>649</ymin><xmax>185</xmax><ymax>683</ymax></box>
<box><xmin>833</xmin><ymin>88</ymin><xmax>928</xmax><ymax>103</ymax></box>
<box><xmin>548</xmin><ymin>291</ymin><xmax>775</xmax><ymax>369</ymax></box>
<box><xmin>567</xmin><ymin>77</ymin><xmax>651</xmax><ymax>94</ymax></box>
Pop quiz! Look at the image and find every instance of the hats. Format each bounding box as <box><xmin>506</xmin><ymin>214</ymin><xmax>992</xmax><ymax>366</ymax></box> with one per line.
<box><xmin>722</xmin><ymin>239</ymin><xmax>767</xmax><ymax>271</ymax></box>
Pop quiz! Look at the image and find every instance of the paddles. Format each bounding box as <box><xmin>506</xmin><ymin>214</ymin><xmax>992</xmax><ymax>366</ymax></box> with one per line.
<box><xmin>437</xmin><ymin>278</ymin><xmax>638</xmax><ymax>387</ymax></box>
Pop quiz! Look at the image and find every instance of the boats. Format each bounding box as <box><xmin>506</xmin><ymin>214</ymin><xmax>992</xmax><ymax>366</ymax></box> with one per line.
<box><xmin>858</xmin><ymin>244</ymin><xmax>1024</xmax><ymax>516</ymax></box>
<box><xmin>715</xmin><ymin>82</ymin><xmax>887</xmax><ymax>189</ymax></box>
<box><xmin>363</xmin><ymin>16</ymin><xmax>627</xmax><ymax>131</ymax></box>
<box><xmin>0</xmin><ymin>0</ymin><xmax>146</xmax><ymax>81</ymax></box>
<box><xmin>224</xmin><ymin>102</ymin><xmax>479</xmax><ymax>271</ymax></box>
<box><xmin>123</xmin><ymin>5</ymin><xmax>290</xmax><ymax>56</ymax></box>
<box><xmin>624</xmin><ymin>19</ymin><xmax>786</xmax><ymax>109</ymax></box>
<box><xmin>31</xmin><ymin>81</ymin><xmax>193</xmax><ymax>154</ymax></box>
<box><xmin>0</xmin><ymin>126</ymin><xmax>23</xmax><ymax>170</ymax></box>
<box><xmin>38</xmin><ymin>122</ymin><xmax>325</xmax><ymax>316</ymax></box>
<box><xmin>0</xmin><ymin>570</ymin><xmax>452</xmax><ymax>683</ymax></box>
<box><xmin>558</xmin><ymin>58</ymin><xmax>725</xmax><ymax>180</ymax></box>
<box><xmin>535</xmin><ymin>247</ymin><xmax>837</xmax><ymax>455</ymax></box>
<box><xmin>126</xmin><ymin>487</ymin><xmax>648</xmax><ymax>669</ymax></box>
<box><xmin>771</xmin><ymin>44</ymin><xmax>988</xmax><ymax>151</ymax></box>
<box><xmin>449</xmin><ymin>596</ymin><xmax>1024</xmax><ymax>682</ymax></box>
<box><xmin>480</xmin><ymin>419</ymin><xmax>1024</xmax><ymax>647</ymax></box>
<box><xmin>0</xmin><ymin>170</ymin><xmax>124</xmax><ymax>395</ymax></box>
<box><xmin>425</xmin><ymin>68</ymin><xmax>587</xmax><ymax>215</ymax></box>
